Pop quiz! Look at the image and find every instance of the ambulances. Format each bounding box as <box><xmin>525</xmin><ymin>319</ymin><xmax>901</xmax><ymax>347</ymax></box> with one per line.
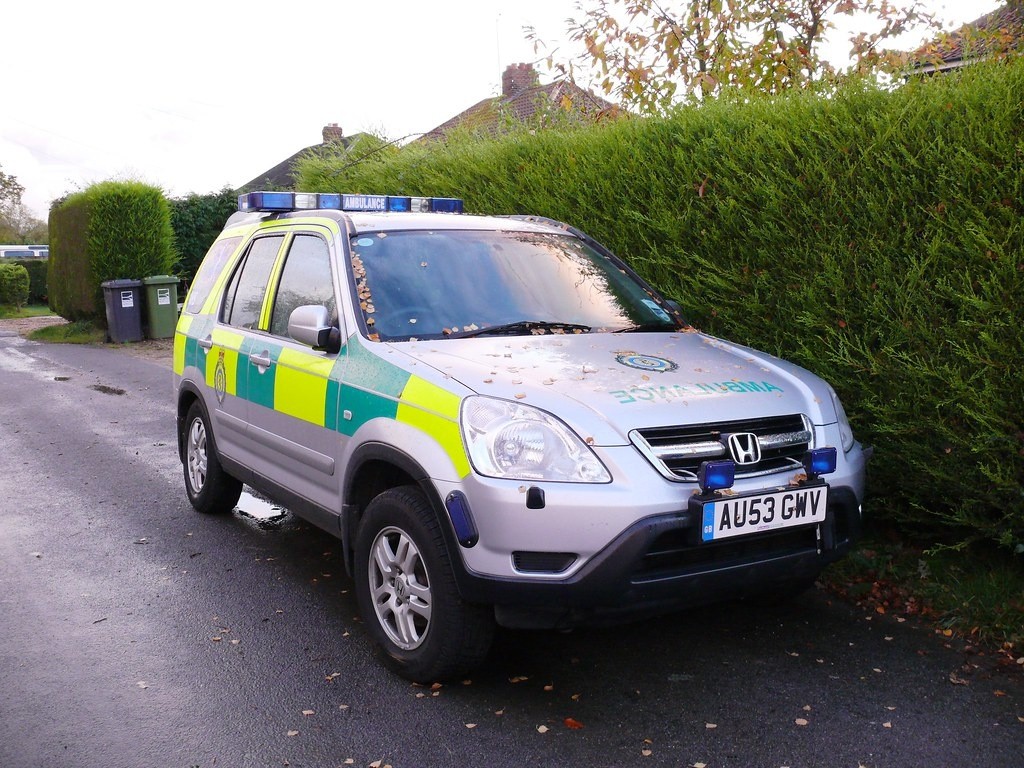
<box><xmin>173</xmin><ymin>190</ymin><xmax>870</xmax><ymax>689</ymax></box>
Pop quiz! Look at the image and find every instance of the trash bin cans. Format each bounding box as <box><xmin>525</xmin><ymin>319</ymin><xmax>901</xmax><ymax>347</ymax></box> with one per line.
<box><xmin>142</xmin><ymin>274</ymin><xmax>181</xmax><ymax>339</ymax></box>
<box><xmin>101</xmin><ymin>279</ymin><xmax>142</xmax><ymax>343</ymax></box>
